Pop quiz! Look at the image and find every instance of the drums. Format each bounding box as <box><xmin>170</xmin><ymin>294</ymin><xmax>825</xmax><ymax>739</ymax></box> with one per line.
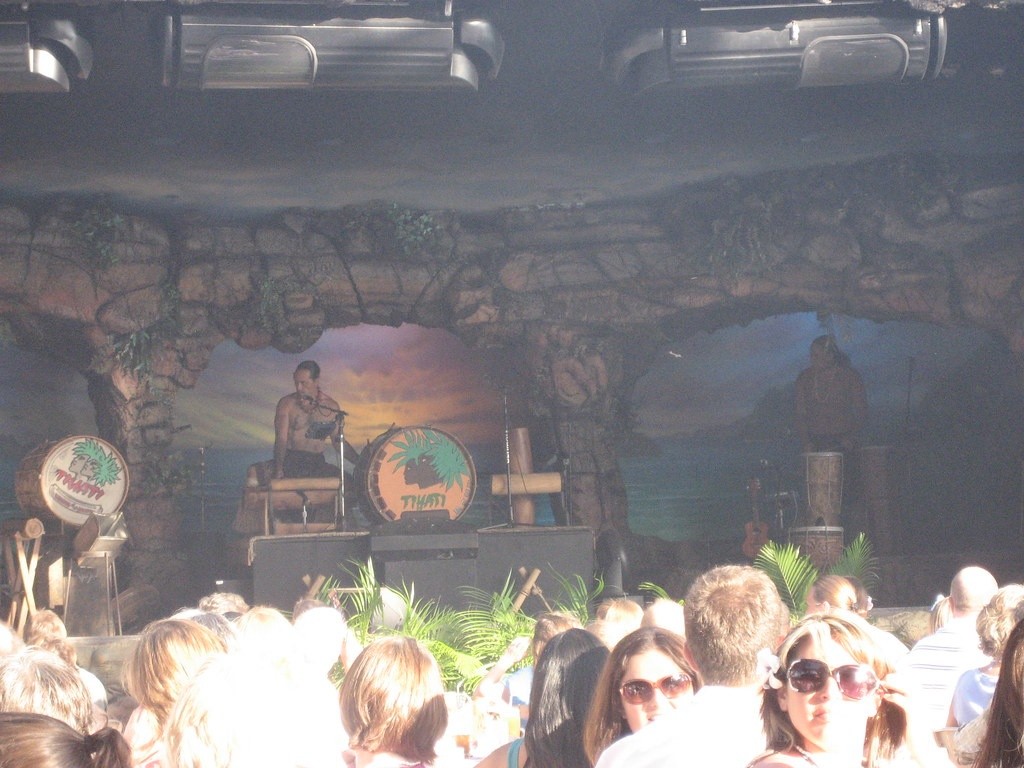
<box><xmin>352</xmin><ymin>425</ymin><xmax>477</xmax><ymax>527</ymax></box>
<box><xmin>860</xmin><ymin>443</ymin><xmax>905</xmax><ymax>558</ymax></box>
<box><xmin>15</xmin><ymin>434</ymin><xmax>131</xmax><ymax>529</ymax></box>
<box><xmin>780</xmin><ymin>451</ymin><xmax>844</xmax><ymax>576</ymax></box>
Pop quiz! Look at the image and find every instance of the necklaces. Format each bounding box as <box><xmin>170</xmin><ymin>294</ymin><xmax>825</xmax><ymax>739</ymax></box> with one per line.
<box><xmin>990</xmin><ymin>659</ymin><xmax>1002</xmax><ymax>665</ymax></box>
<box><xmin>793</xmin><ymin>744</ymin><xmax>821</xmax><ymax>768</ymax></box>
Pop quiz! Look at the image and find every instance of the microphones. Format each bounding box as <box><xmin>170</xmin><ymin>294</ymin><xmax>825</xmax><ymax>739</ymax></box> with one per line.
<box><xmin>303</xmin><ymin>396</ymin><xmax>313</xmax><ymax>401</ymax></box>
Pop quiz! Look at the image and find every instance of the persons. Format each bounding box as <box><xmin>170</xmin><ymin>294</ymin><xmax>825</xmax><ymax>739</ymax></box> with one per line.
<box><xmin>792</xmin><ymin>335</ymin><xmax>868</xmax><ymax>551</ymax></box>
<box><xmin>0</xmin><ymin>564</ymin><xmax>1024</xmax><ymax>768</ymax></box>
<box><xmin>272</xmin><ymin>359</ymin><xmax>360</xmax><ymax>523</ymax></box>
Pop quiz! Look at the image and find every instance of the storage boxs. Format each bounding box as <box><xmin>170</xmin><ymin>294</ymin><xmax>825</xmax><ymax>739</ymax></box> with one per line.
<box><xmin>241</xmin><ymin>476</ymin><xmax>340</xmax><ymax>534</ymax></box>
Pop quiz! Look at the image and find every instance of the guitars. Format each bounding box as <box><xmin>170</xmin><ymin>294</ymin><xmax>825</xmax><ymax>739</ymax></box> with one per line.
<box><xmin>741</xmin><ymin>476</ymin><xmax>770</xmax><ymax>559</ymax></box>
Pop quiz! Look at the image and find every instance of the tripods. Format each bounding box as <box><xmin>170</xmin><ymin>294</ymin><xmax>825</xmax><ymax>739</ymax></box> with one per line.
<box><xmin>481</xmin><ymin>389</ymin><xmax>552</xmax><ymax>530</ymax></box>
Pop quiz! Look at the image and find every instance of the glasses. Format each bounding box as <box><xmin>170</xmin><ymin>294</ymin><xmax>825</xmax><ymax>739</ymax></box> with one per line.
<box><xmin>785</xmin><ymin>657</ymin><xmax>888</xmax><ymax>701</ymax></box>
<box><xmin>618</xmin><ymin>672</ymin><xmax>693</xmax><ymax>706</ymax></box>
